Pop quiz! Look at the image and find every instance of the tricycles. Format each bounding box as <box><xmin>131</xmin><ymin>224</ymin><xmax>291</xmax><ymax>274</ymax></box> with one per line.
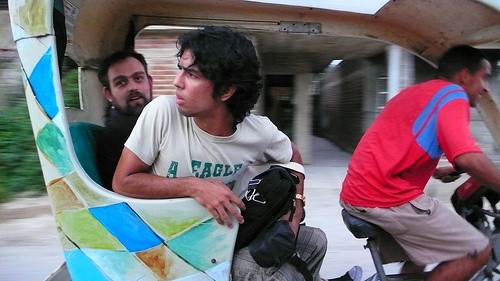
<box><xmin>1</xmin><ymin>0</ymin><xmax>496</xmax><ymax>281</ymax></box>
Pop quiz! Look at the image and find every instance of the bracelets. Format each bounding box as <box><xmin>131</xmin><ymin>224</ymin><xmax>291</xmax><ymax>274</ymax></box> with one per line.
<box><xmin>294</xmin><ymin>191</ymin><xmax>307</xmax><ymax>211</ymax></box>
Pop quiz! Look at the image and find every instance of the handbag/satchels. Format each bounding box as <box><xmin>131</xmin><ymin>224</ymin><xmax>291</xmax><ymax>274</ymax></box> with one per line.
<box><xmin>249</xmin><ymin>218</ymin><xmax>296</xmax><ymax>270</ymax></box>
<box><xmin>234</xmin><ymin>166</ymin><xmax>298</xmax><ymax>251</ymax></box>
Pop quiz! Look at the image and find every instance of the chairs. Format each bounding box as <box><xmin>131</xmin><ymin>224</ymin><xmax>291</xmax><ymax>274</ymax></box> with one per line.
<box><xmin>64</xmin><ymin>64</ymin><xmax>111</xmax><ymax>194</ymax></box>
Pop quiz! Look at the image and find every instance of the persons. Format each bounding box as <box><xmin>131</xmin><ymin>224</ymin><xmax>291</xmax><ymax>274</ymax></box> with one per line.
<box><xmin>109</xmin><ymin>27</ymin><xmax>364</xmax><ymax>280</ymax></box>
<box><xmin>340</xmin><ymin>44</ymin><xmax>496</xmax><ymax>280</ymax></box>
<box><xmin>96</xmin><ymin>47</ymin><xmax>154</xmax><ymax>190</ymax></box>
